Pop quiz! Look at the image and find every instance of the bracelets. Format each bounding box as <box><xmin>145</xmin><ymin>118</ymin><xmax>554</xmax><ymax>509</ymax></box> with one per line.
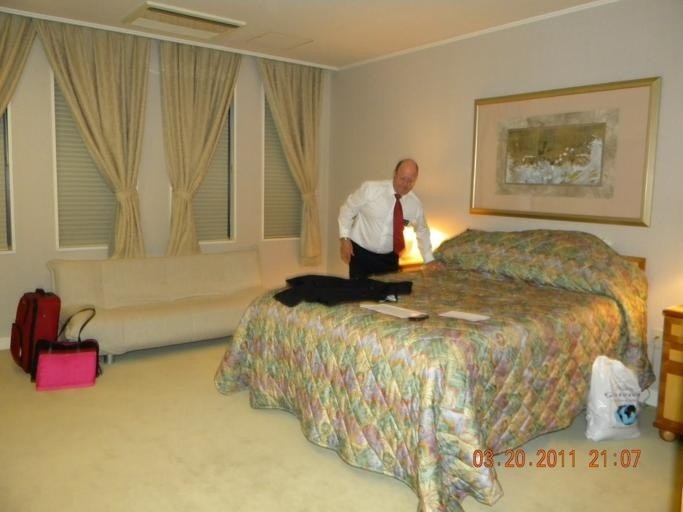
<box><xmin>339</xmin><ymin>237</ymin><xmax>349</xmax><ymax>242</ymax></box>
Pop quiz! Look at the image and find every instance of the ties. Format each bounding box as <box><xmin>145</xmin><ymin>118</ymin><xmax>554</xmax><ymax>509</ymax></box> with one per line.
<box><xmin>392</xmin><ymin>192</ymin><xmax>406</xmax><ymax>255</ymax></box>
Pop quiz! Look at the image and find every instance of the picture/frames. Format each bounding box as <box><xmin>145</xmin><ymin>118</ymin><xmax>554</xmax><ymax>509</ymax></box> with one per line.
<box><xmin>469</xmin><ymin>76</ymin><xmax>665</xmax><ymax>227</ymax></box>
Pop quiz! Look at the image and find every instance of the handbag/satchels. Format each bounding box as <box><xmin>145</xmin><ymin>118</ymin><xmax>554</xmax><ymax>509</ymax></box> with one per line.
<box><xmin>34</xmin><ymin>348</ymin><xmax>97</xmax><ymax>391</ymax></box>
<box><xmin>29</xmin><ymin>305</ymin><xmax>101</xmax><ymax>383</ymax></box>
<box><xmin>584</xmin><ymin>353</ymin><xmax>644</xmax><ymax>443</ymax></box>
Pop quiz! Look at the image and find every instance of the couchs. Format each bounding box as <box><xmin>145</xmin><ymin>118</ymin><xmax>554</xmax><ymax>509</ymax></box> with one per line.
<box><xmin>47</xmin><ymin>249</ymin><xmax>269</xmax><ymax>365</ymax></box>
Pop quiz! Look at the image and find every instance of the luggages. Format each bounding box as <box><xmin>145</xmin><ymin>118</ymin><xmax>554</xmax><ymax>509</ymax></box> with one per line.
<box><xmin>9</xmin><ymin>288</ymin><xmax>61</xmax><ymax>374</ymax></box>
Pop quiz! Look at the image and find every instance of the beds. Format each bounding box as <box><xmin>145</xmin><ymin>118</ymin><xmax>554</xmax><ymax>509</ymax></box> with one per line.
<box><xmin>214</xmin><ymin>228</ymin><xmax>654</xmax><ymax>486</ymax></box>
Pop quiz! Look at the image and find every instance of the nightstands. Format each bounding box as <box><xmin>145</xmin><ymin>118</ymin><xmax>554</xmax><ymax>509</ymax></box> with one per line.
<box><xmin>652</xmin><ymin>304</ymin><xmax>683</xmax><ymax>442</ymax></box>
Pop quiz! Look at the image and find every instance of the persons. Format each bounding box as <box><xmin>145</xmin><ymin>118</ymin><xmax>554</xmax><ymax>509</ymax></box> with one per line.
<box><xmin>336</xmin><ymin>158</ymin><xmax>444</xmax><ymax>282</ymax></box>
